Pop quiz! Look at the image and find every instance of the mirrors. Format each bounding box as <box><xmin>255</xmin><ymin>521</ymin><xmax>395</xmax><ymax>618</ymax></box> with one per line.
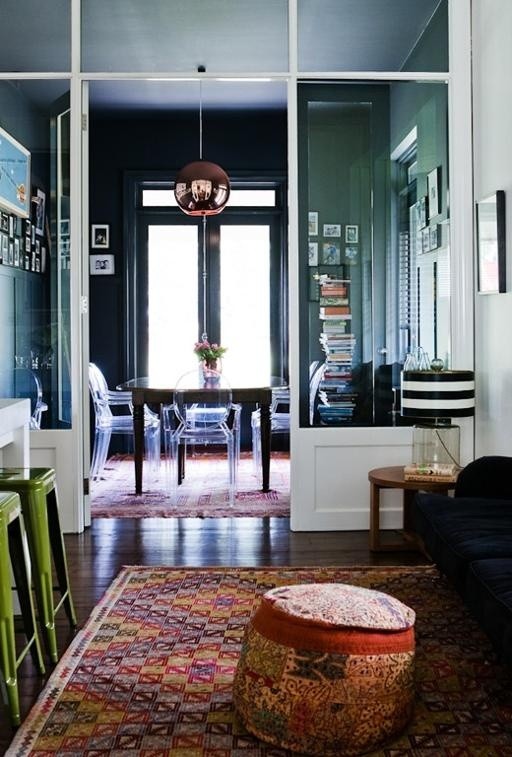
<box><xmin>301</xmin><ymin>80</ymin><xmax>452</xmax><ymax>432</ymax></box>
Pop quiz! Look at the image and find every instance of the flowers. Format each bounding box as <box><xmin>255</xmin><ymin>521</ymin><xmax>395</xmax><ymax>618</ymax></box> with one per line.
<box><xmin>194</xmin><ymin>341</ymin><xmax>225</xmax><ymax>368</ymax></box>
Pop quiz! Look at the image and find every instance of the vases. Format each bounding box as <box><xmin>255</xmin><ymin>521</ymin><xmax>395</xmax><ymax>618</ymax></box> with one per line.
<box><xmin>202</xmin><ymin>358</ymin><xmax>222</xmax><ymax>388</ymax></box>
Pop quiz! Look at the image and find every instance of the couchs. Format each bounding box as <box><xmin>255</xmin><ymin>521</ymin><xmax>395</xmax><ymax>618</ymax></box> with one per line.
<box><xmin>412</xmin><ymin>456</ymin><xmax>512</xmax><ymax>657</ymax></box>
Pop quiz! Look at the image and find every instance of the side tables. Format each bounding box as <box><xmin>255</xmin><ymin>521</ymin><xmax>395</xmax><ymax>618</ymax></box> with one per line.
<box><xmin>368</xmin><ymin>466</ymin><xmax>463</xmax><ymax>554</ymax></box>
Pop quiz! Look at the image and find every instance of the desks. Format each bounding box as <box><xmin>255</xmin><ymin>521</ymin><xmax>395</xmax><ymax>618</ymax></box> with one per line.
<box><xmin>116</xmin><ymin>376</ymin><xmax>272</xmax><ymax>496</ymax></box>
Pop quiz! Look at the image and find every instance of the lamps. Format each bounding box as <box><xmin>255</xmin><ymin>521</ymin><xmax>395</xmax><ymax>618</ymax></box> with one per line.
<box><xmin>400</xmin><ymin>359</ymin><xmax>475</xmax><ymax>469</ymax></box>
<box><xmin>173</xmin><ymin>65</ymin><xmax>230</xmax><ymax>217</ymax></box>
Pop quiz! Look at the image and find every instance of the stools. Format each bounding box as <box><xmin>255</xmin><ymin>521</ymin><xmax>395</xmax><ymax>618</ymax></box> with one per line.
<box><xmin>0</xmin><ymin>492</ymin><xmax>46</xmax><ymax>727</ymax></box>
<box><xmin>0</xmin><ymin>466</ymin><xmax>77</xmax><ymax>666</ymax></box>
<box><xmin>230</xmin><ymin>583</ymin><xmax>416</xmax><ymax>756</ymax></box>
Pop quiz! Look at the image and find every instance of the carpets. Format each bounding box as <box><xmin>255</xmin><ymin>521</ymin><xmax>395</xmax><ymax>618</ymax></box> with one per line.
<box><xmin>91</xmin><ymin>452</ymin><xmax>290</xmax><ymax>520</ymax></box>
<box><xmin>5</xmin><ymin>562</ymin><xmax>512</xmax><ymax>757</ymax></box>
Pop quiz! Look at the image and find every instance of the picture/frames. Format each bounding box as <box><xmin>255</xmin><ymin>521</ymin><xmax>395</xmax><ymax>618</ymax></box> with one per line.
<box><xmin>24</xmin><ymin>235</ymin><xmax>31</xmax><ymax>253</ymax></box>
<box><xmin>24</xmin><ymin>255</ymin><xmax>29</xmax><ymax>270</ymax></box>
<box><xmin>323</xmin><ymin>224</ymin><xmax>341</xmax><ymax>238</ymax></box>
<box><xmin>475</xmin><ymin>190</ymin><xmax>505</xmax><ymax>295</ymax></box>
<box><xmin>323</xmin><ymin>242</ymin><xmax>341</xmax><ymax>264</ymax></box>
<box><xmin>0</xmin><ymin>128</ymin><xmax>31</xmax><ymax>219</ymax></box>
<box><xmin>91</xmin><ymin>224</ymin><xmax>110</xmax><ymax>248</ymax></box>
<box><xmin>61</xmin><ymin>220</ymin><xmax>70</xmax><ymax>236</ymax></box>
<box><xmin>41</xmin><ymin>247</ymin><xmax>48</xmax><ymax>273</ymax></box>
<box><xmin>308</xmin><ymin>211</ymin><xmax>318</xmax><ymax>236</ymax></box>
<box><xmin>32</xmin><ymin>225</ymin><xmax>36</xmax><ymax>245</ymax></box>
<box><xmin>0</xmin><ymin>210</ymin><xmax>9</xmax><ymax>233</ymax></box>
<box><xmin>426</xmin><ymin>167</ymin><xmax>440</xmax><ymax>220</ymax></box>
<box><xmin>415</xmin><ymin>223</ymin><xmax>440</xmax><ymax>255</ymax></box>
<box><xmin>308</xmin><ymin>241</ymin><xmax>318</xmax><ymax>267</ymax></box>
<box><xmin>414</xmin><ymin>196</ymin><xmax>427</xmax><ymax>232</ymax></box>
<box><xmin>345</xmin><ymin>225</ymin><xmax>359</xmax><ymax>243</ymax></box>
<box><xmin>34</xmin><ymin>188</ymin><xmax>46</xmax><ymax>237</ymax></box>
<box><xmin>2</xmin><ymin>248</ymin><xmax>8</xmax><ymax>265</ymax></box>
<box><xmin>9</xmin><ymin>243</ymin><xmax>13</xmax><ymax>265</ymax></box>
<box><xmin>14</xmin><ymin>238</ymin><xmax>19</xmax><ymax>267</ymax></box>
<box><xmin>30</xmin><ymin>251</ymin><xmax>36</xmax><ymax>272</ymax></box>
<box><xmin>24</xmin><ymin>218</ymin><xmax>32</xmax><ymax>236</ymax></box>
<box><xmin>89</xmin><ymin>254</ymin><xmax>115</xmax><ymax>275</ymax></box>
<box><xmin>344</xmin><ymin>246</ymin><xmax>359</xmax><ymax>266</ymax></box>
<box><xmin>36</xmin><ymin>240</ymin><xmax>40</xmax><ymax>255</ymax></box>
<box><xmin>8</xmin><ymin>216</ymin><xmax>13</xmax><ymax>239</ymax></box>
<box><xmin>35</xmin><ymin>258</ymin><xmax>41</xmax><ymax>273</ymax></box>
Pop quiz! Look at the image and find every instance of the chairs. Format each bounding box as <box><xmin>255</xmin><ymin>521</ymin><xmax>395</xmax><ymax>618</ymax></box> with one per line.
<box><xmin>162</xmin><ymin>403</ymin><xmax>241</xmax><ymax>472</ymax></box>
<box><xmin>87</xmin><ymin>364</ymin><xmax>161</xmax><ymax>486</ymax></box>
<box><xmin>251</xmin><ymin>387</ymin><xmax>290</xmax><ymax>484</ymax></box>
<box><xmin>170</xmin><ymin>368</ymin><xmax>235</xmax><ymax>508</ymax></box>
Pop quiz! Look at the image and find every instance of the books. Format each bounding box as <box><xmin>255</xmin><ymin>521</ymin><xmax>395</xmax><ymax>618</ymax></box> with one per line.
<box><xmin>312</xmin><ymin>274</ymin><xmax>359</xmax><ymax>426</ymax></box>
<box><xmin>403</xmin><ymin>463</ymin><xmax>460</xmax><ymax>483</ymax></box>
